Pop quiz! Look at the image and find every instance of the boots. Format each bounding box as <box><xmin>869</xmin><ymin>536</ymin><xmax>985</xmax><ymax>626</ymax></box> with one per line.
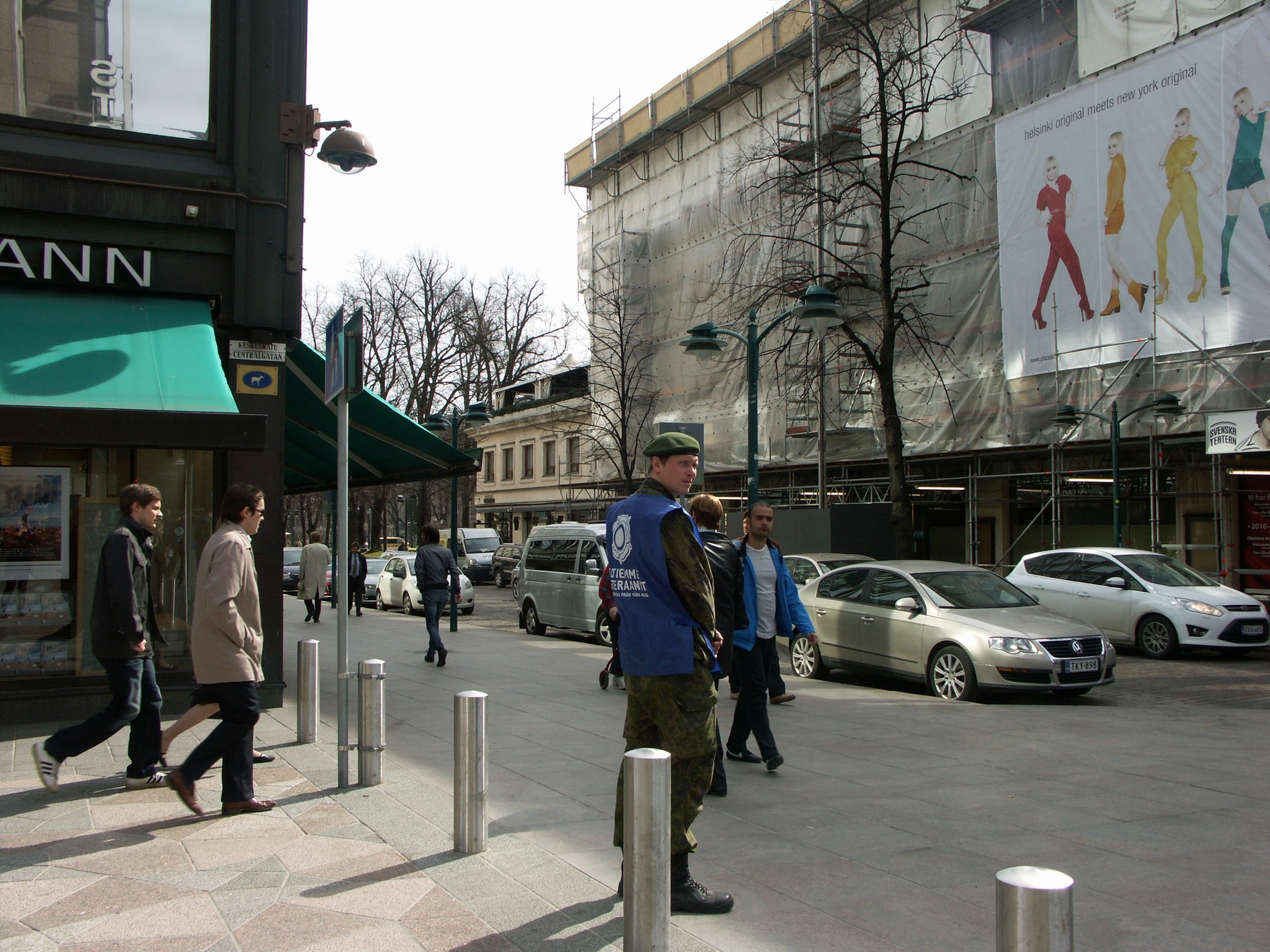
<box><xmin>670</xmin><ymin>851</ymin><xmax>734</xmax><ymax>914</ymax></box>
<box><xmin>618</xmin><ymin>847</ymin><xmax>623</xmax><ymax>897</ymax></box>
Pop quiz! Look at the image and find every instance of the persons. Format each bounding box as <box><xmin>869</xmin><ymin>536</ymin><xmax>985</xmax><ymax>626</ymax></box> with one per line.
<box><xmin>1099</xmin><ymin>131</ymin><xmax>1149</xmax><ymax>316</ymax></box>
<box><xmin>32</xmin><ymin>483</ymin><xmax>166</xmax><ymax>792</ymax></box>
<box><xmin>600</xmin><ymin>430</ymin><xmax>736</xmax><ymax>911</ymax></box>
<box><xmin>1154</xmin><ymin>107</ymin><xmax>1207</xmax><ymax>302</ymax></box>
<box><xmin>680</xmin><ymin>490</ymin><xmax>815</xmax><ymax>769</ymax></box>
<box><xmin>343</xmin><ymin>541</ymin><xmax>368</xmax><ymax>616</ymax></box>
<box><xmin>398</xmin><ymin>541</ymin><xmax>409</xmax><ymax>551</ymax></box>
<box><xmin>414</xmin><ymin>526</ymin><xmax>461</xmax><ymax>667</ymax></box>
<box><xmin>596</xmin><ymin>562</ymin><xmax>629</xmax><ymax>691</ymax></box>
<box><xmin>164</xmin><ymin>484</ymin><xmax>276</xmax><ymax>818</ymax></box>
<box><xmin>1031</xmin><ymin>157</ymin><xmax>1094</xmax><ymax>329</ymax></box>
<box><xmin>1208</xmin><ymin>87</ymin><xmax>1270</xmax><ymax>296</ymax></box>
<box><xmin>160</xmin><ymin>670</ymin><xmax>275</xmax><ymax>769</ymax></box>
<box><xmin>1235</xmin><ymin>410</ymin><xmax>1270</xmax><ymax>453</ymax></box>
<box><xmin>297</xmin><ymin>531</ymin><xmax>332</xmax><ymax>623</ymax></box>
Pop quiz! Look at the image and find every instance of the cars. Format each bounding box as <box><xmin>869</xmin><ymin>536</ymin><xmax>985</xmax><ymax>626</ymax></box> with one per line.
<box><xmin>1001</xmin><ymin>544</ymin><xmax>1269</xmax><ymax>660</ymax></box>
<box><xmin>283</xmin><ymin>547</ymin><xmax>304</xmax><ymax>591</ymax></box>
<box><xmin>780</xmin><ymin>553</ymin><xmax>879</xmax><ymax>591</ymax></box>
<box><xmin>510</xmin><ymin>560</ymin><xmax>519</xmax><ymax>601</ymax></box>
<box><xmin>788</xmin><ymin>559</ymin><xmax>1118</xmax><ymax>702</ymax></box>
<box><xmin>324</xmin><ymin>549</ymin><xmax>418</xmax><ymax>600</ymax></box>
<box><xmin>375</xmin><ymin>554</ymin><xmax>475</xmax><ymax>615</ymax></box>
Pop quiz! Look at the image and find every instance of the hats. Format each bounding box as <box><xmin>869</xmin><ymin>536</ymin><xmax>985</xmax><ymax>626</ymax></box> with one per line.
<box><xmin>642</xmin><ymin>432</ymin><xmax>700</xmax><ymax>458</ymax></box>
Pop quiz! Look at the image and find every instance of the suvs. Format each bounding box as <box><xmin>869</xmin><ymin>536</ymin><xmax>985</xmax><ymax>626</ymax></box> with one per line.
<box><xmin>489</xmin><ymin>543</ymin><xmax>526</xmax><ymax>588</ymax></box>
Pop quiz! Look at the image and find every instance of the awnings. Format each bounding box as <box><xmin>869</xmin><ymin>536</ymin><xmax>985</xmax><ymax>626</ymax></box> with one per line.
<box><xmin>0</xmin><ymin>283</ymin><xmax>240</xmax><ymax>419</ymax></box>
<box><xmin>281</xmin><ymin>338</ymin><xmax>481</xmax><ymax>492</ymax></box>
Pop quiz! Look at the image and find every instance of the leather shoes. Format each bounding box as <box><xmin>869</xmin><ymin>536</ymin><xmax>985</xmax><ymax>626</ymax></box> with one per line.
<box><xmin>222</xmin><ymin>797</ymin><xmax>277</xmax><ymax>815</ymax></box>
<box><xmin>165</xmin><ymin>768</ymin><xmax>205</xmax><ymax>816</ymax></box>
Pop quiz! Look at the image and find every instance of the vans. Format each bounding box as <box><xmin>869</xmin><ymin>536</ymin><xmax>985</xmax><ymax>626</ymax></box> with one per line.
<box><xmin>380</xmin><ymin>536</ymin><xmax>410</xmax><ymax>551</ymax></box>
<box><xmin>516</xmin><ymin>521</ymin><xmax>613</xmax><ymax>647</ymax></box>
<box><xmin>438</xmin><ymin>527</ymin><xmax>504</xmax><ymax>583</ymax></box>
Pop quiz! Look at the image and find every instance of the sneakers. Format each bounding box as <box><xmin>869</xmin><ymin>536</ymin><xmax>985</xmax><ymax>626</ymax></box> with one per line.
<box><xmin>612</xmin><ymin>674</ymin><xmax>626</xmax><ymax>689</ymax></box>
<box><xmin>766</xmin><ymin>754</ymin><xmax>783</xmax><ymax>770</ymax></box>
<box><xmin>125</xmin><ymin>771</ymin><xmax>169</xmax><ymax>789</ymax></box>
<box><xmin>726</xmin><ymin>747</ymin><xmax>762</xmax><ymax>764</ymax></box>
<box><xmin>769</xmin><ymin>694</ymin><xmax>796</xmax><ymax>704</ymax></box>
<box><xmin>32</xmin><ymin>741</ymin><xmax>61</xmax><ymax>793</ymax></box>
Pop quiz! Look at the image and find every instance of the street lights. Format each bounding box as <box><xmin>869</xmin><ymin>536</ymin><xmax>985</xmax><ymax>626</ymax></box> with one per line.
<box><xmin>420</xmin><ymin>401</ymin><xmax>496</xmax><ymax>631</ymax></box>
<box><xmin>676</xmin><ymin>283</ymin><xmax>851</xmax><ymax>511</ymax></box>
<box><xmin>394</xmin><ymin>494</ymin><xmax>408</xmax><ymax>550</ymax></box>
<box><xmin>1049</xmin><ymin>393</ymin><xmax>1188</xmax><ymax>548</ymax></box>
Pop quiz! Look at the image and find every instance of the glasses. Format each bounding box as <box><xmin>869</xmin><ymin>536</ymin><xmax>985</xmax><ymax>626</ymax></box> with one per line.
<box><xmin>422</xmin><ymin>534</ymin><xmax>425</xmax><ymax>537</ymax></box>
<box><xmin>251</xmin><ymin>507</ymin><xmax>266</xmax><ymax>517</ymax></box>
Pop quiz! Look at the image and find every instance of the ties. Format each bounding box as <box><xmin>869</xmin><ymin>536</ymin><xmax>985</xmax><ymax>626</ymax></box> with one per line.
<box><xmin>353</xmin><ymin>555</ymin><xmax>357</xmax><ymax>578</ymax></box>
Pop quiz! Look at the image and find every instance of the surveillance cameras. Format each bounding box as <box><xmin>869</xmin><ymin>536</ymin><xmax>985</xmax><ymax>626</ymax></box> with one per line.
<box><xmin>316</xmin><ymin>127</ymin><xmax>377</xmax><ymax>175</ymax></box>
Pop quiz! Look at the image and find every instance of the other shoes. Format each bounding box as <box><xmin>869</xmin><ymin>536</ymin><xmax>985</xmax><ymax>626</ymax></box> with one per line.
<box><xmin>706</xmin><ymin>788</ymin><xmax>727</xmax><ymax>797</ymax></box>
<box><xmin>348</xmin><ymin>611</ymin><xmax>350</xmax><ymax>615</ymax></box>
<box><xmin>155</xmin><ymin>663</ymin><xmax>180</xmax><ymax>671</ymax></box>
<box><xmin>730</xmin><ymin>693</ymin><xmax>739</xmax><ymax>700</ymax></box>
<box><xmin>159</xmin><ymin>752</ymin><xmax>167</xmax><ymax>768</ymax></box>
<box><xmin>356</xmin><ymin>612</ymin><xmax>363</xmax><ymax>616</ymax></box>
<box><xmin>305</xmin><ymin>611</ymin><xmax>315</xmax><ymax>622</ymax></box>
<box><xmin>437</xmin><ymin>647</ymin><xmax>447</xmax><ymax>666</ymax></box>
<box><xmin>253</xmin><ymin>754</ymin><xmax>275</xmax><ymax>763</ymax></box>
<box><xmin>425</xmin><ymin>654</ymin><xmax>435</xmax><ymax>662</ymax></box>
<box><xmin>314</xmin><ymin>619</ymin><xmax>320</xmax><ymax>623</ymax></box>
